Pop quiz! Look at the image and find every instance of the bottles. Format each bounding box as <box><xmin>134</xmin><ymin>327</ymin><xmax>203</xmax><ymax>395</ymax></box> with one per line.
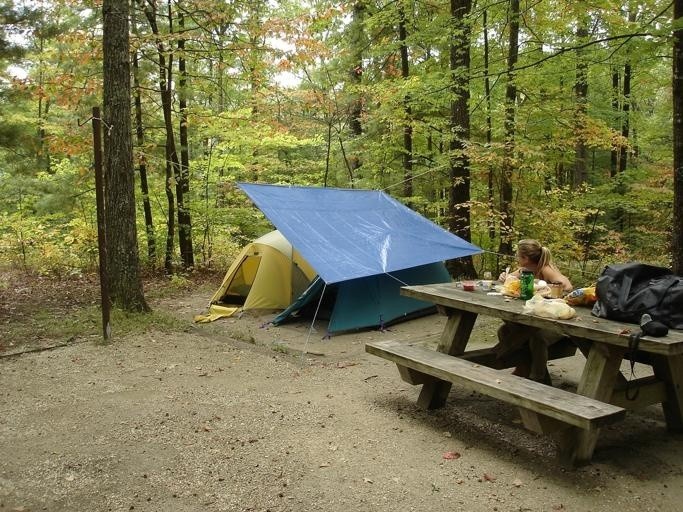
<box><xmin>520</xmin><ymin>271</ymin><xmax>534</xmax><ymax>301</ymax></box>
<box><xmin>552</xmin><ymin>281</ymin><xmax>563</xmax><ymax>299</ymax></box>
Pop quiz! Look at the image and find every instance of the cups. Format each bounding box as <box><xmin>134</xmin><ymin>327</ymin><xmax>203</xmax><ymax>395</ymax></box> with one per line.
<box><xmin>482</xmin><ymin>281</ymin><xmax>491</xmax><ymax>292</ymax></box>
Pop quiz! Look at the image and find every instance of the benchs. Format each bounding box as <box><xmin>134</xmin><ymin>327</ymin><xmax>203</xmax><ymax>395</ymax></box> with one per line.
<box><xmin>365</xmin><ymin>339</ymin><xmax>626</xmax><ymax>466</ymax></box>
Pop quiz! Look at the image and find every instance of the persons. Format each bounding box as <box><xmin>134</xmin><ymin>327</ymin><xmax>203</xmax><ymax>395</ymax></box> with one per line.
<box><xmin>193</xmin><ymin>228</ymin><xmax>318</xmax><ymax>324</ymax></box>
<box><xmin>498</xmin><ymin>239</ymin><xmax>574</xmax><ymax>385</ymax></box>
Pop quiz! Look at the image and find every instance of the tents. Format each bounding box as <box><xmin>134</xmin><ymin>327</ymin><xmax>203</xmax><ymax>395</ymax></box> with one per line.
<box><xmin>271</xmin><ymin>259</ymin><xmax>454</xmax><ymax>337</ymax></box>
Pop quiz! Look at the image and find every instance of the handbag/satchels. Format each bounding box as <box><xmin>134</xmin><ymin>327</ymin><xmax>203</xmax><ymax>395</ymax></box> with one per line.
<box><xmin>590</xmin><ymin>259</ymin><xmax>683</xmax><ymax>332</ymax></box>
